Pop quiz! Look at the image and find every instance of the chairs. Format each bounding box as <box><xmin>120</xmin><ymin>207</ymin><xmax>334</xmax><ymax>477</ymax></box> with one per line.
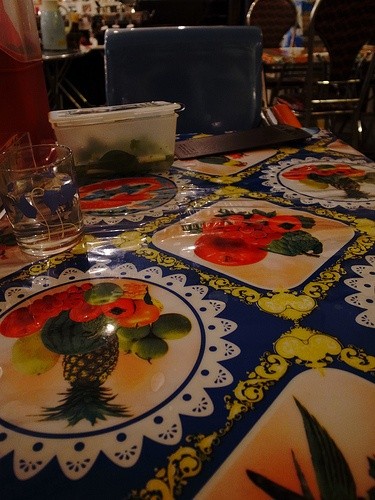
<box><xmin>103</xmin><ymin>26</ymin><xmax>263</xmax><ymax>141</ymax></box>
<box><xmin>244</xmin><ymin>0</ymin><xmax>375</xmax><ymax>163</ymax></box>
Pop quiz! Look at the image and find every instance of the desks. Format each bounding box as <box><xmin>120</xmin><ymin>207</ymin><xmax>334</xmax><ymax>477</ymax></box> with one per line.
<box><xmin>261</xmin><ymin>44</ymin><xmax>375</xmax><ymax>110</ymax></box>
<box><xmin>0</xmin><ymin>127</ymin><xmax>375</xmax><ymax>500</ymax></box>
<box><xmin>40</xmin><ymin>46</ymin><xmax>92</xmax><ymax>111</ymax></box>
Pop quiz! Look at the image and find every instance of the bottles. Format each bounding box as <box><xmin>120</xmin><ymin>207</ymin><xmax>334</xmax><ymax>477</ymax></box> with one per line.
<box><xmin>41</xmin><ymin>0</ymin><xmax>67</xmax><ymax>52</ymax></box>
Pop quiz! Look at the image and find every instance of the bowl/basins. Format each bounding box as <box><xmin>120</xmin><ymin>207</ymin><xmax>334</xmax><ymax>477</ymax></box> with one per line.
<box><xmin>47</xmin><ymin>101</ymin><xmax>181</xmax><ymax>169</ymax></box>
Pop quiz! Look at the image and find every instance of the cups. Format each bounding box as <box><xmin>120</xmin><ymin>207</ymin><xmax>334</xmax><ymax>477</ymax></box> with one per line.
<box><xmin>0</xmin><ymin>144</ymin><xmax>85</xmax><ymax>256</ymax></box>
